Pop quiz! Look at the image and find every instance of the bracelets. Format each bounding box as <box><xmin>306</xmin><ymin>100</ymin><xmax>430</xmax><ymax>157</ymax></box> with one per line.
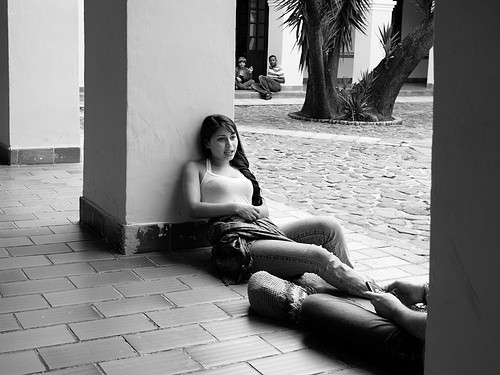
<box><xmin>422</xmin><ymin>286</ymin><xmax>427</xmax><ymax>301</ymax></box>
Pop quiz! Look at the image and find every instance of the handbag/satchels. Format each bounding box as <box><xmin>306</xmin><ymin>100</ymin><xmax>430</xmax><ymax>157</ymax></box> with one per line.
<box><xmin>248</xmin><ymin>271</ymin><xmax>316</xmax><ymax>322</ymax></box>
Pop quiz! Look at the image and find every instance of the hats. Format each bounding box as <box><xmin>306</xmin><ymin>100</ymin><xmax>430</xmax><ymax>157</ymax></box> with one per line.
<box><xmin>238</xmin><ymin>57</ymin><xmax>246</xmax><ymax>61</ymax></box>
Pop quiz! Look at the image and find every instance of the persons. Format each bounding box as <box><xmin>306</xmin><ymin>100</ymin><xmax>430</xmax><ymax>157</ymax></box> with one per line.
<box><xmin>235</xmin><ymin>57</ymin><xmax>255</xmax><ymax>90</ymax></box>
<box><xmin>182</xmin><ymin>113</ymin><xmax>383</xmax><ymax>299</ymax></box>
<box><xmin>299</xmin><ymin>280</ymin><xmax>430</xmax><ymax>375</ymax></box>
<box><xmin>251</xmin><ymin>55</ymin><xmax>285</xmax><ymax>100</ymax></box>
